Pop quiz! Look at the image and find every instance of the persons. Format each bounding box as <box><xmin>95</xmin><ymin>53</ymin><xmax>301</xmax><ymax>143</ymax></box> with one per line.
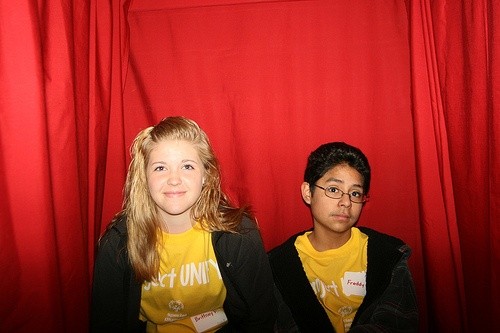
<box><xmin>84</xmin><ymin>113</ymin><xmax>276</xmax><ymax>333</ymax></box>
<box><xmin>262</xmin><ymin>141</ymin><xmax>425</xmax><ymax>333</ymax></box>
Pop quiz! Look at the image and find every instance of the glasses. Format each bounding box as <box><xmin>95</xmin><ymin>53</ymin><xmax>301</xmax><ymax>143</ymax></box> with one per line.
<box><xmin>310</xmin><ymin>180</ymin><xmax>368</xmax><ymax>204</ymax></box>
